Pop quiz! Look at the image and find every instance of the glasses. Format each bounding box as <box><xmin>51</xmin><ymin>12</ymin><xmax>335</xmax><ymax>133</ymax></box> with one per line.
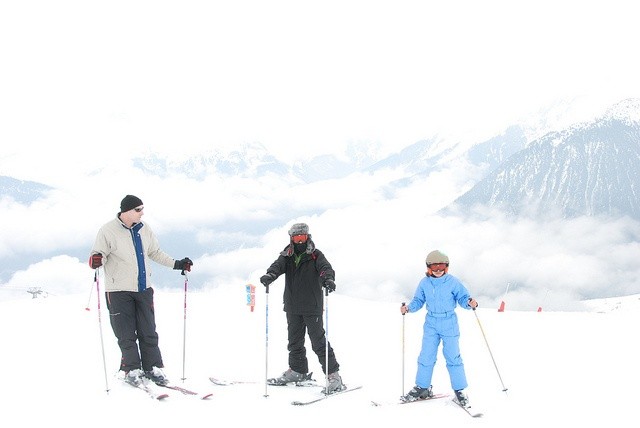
<box><xmin>293</xmin><ymin>233</ymin><xmax>308</xmax><ymax>242</ymax></box>
<box><xmin>134</xmin><ymin>207</ymin><xmax>144</xmax><ymax>212</ymax></box>
<box><xmin>427</xmin><ymin>263</ymin><xmax>447</xmax><ymax>271</ymax></box>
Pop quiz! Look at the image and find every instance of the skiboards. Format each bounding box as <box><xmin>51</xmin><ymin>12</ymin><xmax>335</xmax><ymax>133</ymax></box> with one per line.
<box><xmin>209</xmin><ymin>377</ymin><xmax>363</xmax><ymax>406</ymax></box>
<box><xmin>400</xmin><ymin>385</ymin><xmax>483</xmax><ymax>418</ymax></box>
<box><xmin>120</xmin><ymin>378</ymin><xmax>213</xmax><ymax>401</ymax></box>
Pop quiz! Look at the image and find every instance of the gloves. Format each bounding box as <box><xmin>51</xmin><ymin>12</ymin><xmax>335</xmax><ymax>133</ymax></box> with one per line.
<box><xmin>260</xmin><ymin>273</ymin><xmax>277</xmax><ymax>285</ymax></box>
<box><xmin>89</xmin><ymin>253</ymin><xmax>103</xmax><ymax>268</ymax></box>
<box><xmin>173</xmin><ymin>257</ymin><xmax>193</xmax><ymax>272</ymax></box>
<box><xmin>323</xmin><ymin>277</ymin><xmax>336</xmax><ymax>291</ymax></box>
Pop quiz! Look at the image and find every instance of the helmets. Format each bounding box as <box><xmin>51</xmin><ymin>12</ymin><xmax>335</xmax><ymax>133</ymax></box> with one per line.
<box><xmin>425</xmin><ymin>250</ymin><xmax>450</xmax><ymax>277</ymax></box>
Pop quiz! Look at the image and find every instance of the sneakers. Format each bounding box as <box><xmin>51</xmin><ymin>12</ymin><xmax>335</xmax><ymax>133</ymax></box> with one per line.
<box><xmin>322</xmin><ymin>370</ymin><xmax>343</xmax><ymax>389</ymax></box>
<box><xmin>407</xmin><ymin>385</ymin><xmax>431</xmax><ymax>399</ymax></box>
<box><xmin>143</xmin><ymin>366</ymin><xmax>168</xmax><ymax>385</ymax></box>
<box><xmin>124</xmin><ymin>369</ymin><xmax>147</xmax><ymax>386</ymax></box>
<box><xmin>277</xmin><ymin>368</ymin><xmax>309</xmax><ymax>383</ymax></box>
<box><xmin>455</xmin><ymin>390</ymin><xmax>468</xmax><ymax>406</ymax></box>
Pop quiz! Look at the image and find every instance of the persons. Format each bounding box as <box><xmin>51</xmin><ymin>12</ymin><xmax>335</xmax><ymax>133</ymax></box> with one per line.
<box><xmin>89</xmin><ymin>195</ymin><xmax>193</xmax><ymax>388</ymax></box>
<box><xmin>260</xmin><ymin>223</ymin><xmax>343</xmax><ymax>393</ymax></box>
<box><xmin>400</xmin><ymin>250</ymin><xmax>479</xmax><ymax>406</ymax></box>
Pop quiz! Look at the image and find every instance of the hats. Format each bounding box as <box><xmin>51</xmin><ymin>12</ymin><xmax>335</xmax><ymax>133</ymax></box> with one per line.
<box><xmin>288</xmin><ymin>223</ymin><xmax>309</xmax><ymax>242</ymax></box>
<box><xmin>120</xmin><ymin>194</ymin><xmax>143</xmax><ymax>213</ymax></box>
